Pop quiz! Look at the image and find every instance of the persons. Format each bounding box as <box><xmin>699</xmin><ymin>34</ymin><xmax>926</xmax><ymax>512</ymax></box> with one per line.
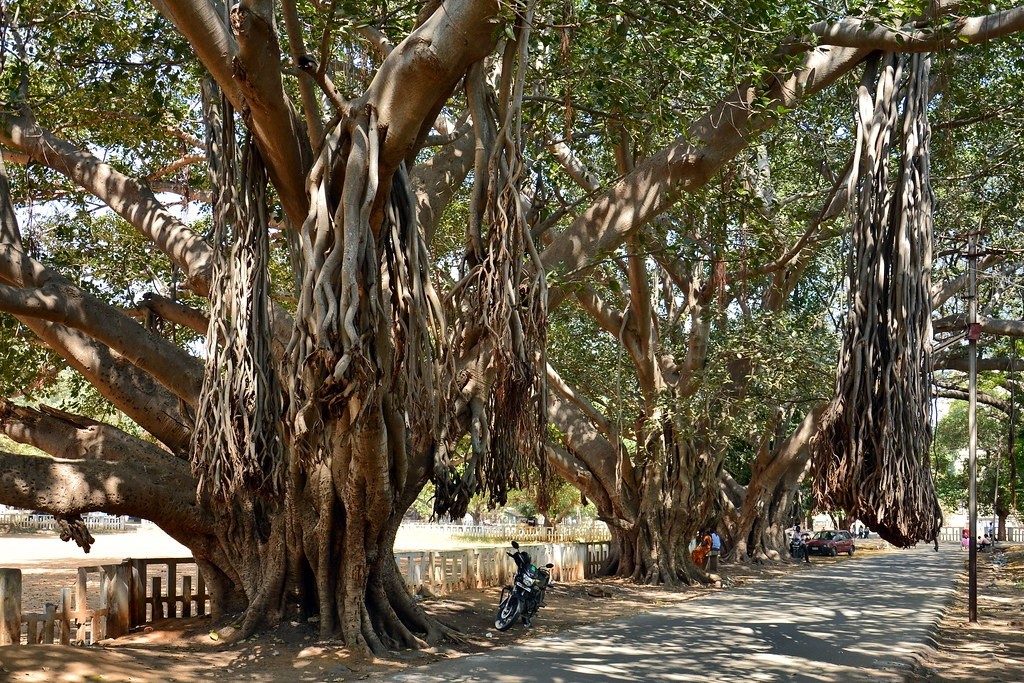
<box><xmin>988</xmin><ymin>522</ymin><xmax>998</xmax><ymax>543</ymax></box>
<box><xmin>791</xmin><ymin>525</ymin><xmax>810</xmax><ymax>563</ymax></box>
<box><xmin>977</xmin><ymin>536</ymin><xmax>982</xmax><ymax>551</ymax></box>
<box><xmin>852</xmin><ymin>524</ymin><xmax>869</xmax><ymax>538</ymax></box>
<box><xmin>982</xmin><ymin>533</ymin><xmax>991</xmax><ymax>548</ymax></box>
<box><xmin>821</xmin><ymin>527</ymin><xmax>843</xmax><ymax>540</ymax></box>
<box><xmin>692</xmin><ymin>528</ymin><xmax>720</xmax><ymax>567</ymax></box>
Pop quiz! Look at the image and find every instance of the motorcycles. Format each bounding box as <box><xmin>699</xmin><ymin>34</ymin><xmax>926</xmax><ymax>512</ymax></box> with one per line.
<box><xmin>494</xmin><ymin>541</ymin><xmax>555</xmax><ymax>632</ymax></box>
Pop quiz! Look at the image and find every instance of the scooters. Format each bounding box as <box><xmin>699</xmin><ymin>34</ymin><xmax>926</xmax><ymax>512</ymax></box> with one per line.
<box><xmin>787</xmin><ymin>533</ymin><xmax>803</xmax><ymax>558</ymax></box>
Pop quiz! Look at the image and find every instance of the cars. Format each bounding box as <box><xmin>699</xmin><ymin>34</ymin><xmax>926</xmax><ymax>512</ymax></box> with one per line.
<box><xmin>806</xmin><ymin>530</ymin><xmax>856</xmax><ymax>557</ymax></box>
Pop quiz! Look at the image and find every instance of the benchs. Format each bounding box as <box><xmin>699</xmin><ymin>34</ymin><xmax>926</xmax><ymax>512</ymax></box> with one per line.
<box><xmin>706</xmin><ymin>549</ymin><xmax>721</xmax><ymax>573</ymax></box>
